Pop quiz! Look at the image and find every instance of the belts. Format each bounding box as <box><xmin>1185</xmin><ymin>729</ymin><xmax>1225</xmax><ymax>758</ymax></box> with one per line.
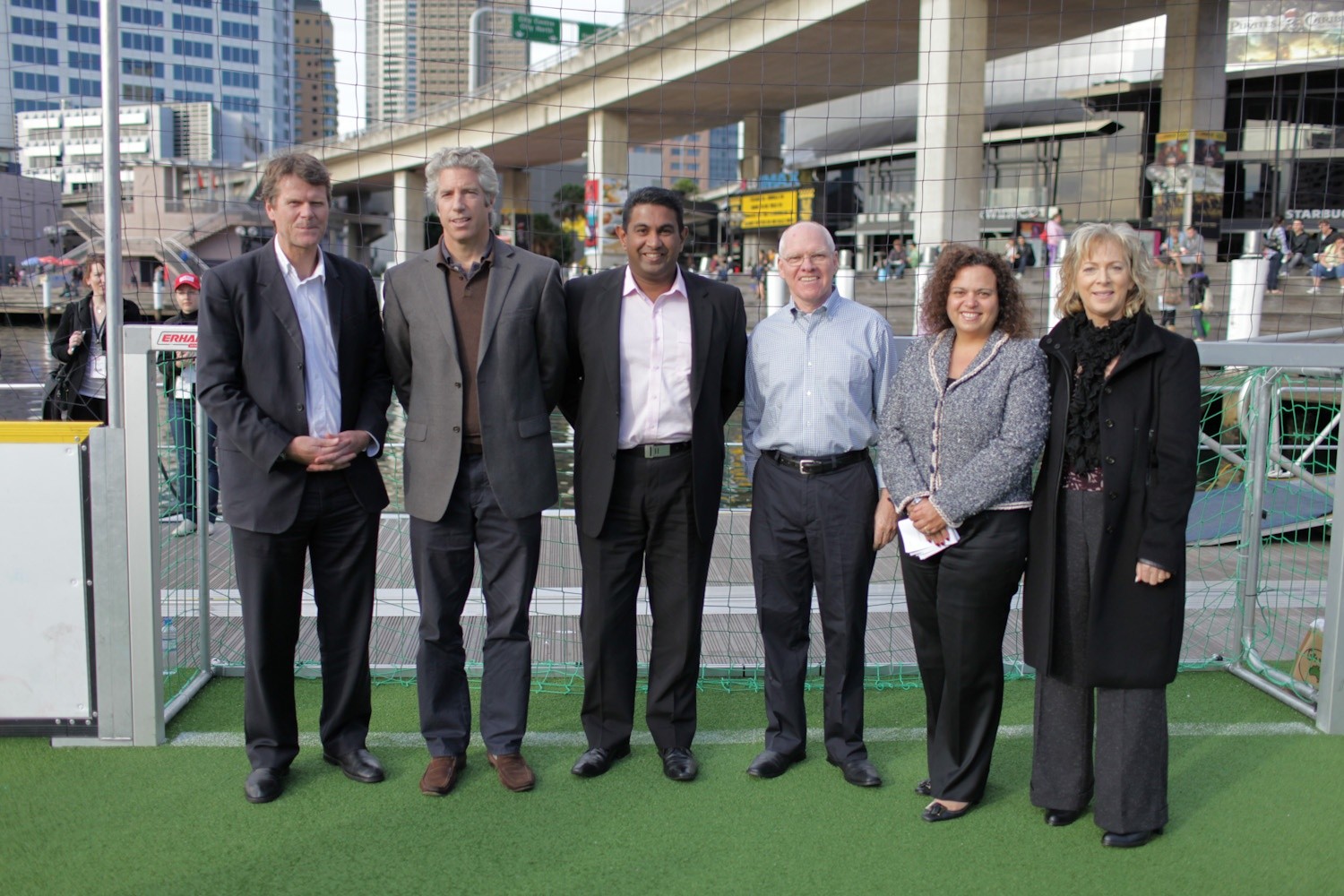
<box><xmin>610</xmin><ymin>441</ymin><xmax>691</xmax><ymax>460</ymax></box>
<box><xmin>764</xmin><ymin>447</ymin><xmax>872</xmax><ymax>477</ymax></box>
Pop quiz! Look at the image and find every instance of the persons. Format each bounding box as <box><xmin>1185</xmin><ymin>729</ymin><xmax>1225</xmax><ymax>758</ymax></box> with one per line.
<box><xmin>51</xmin><ymin>252</ymin><xmax>143</xmax><ymax>426</ymax></box>
<box><xmin>877</xmin><ymin>240</ymin><xmax>1047</xmax><ymax>821</ymax></box>
<box><xmin>1156</xmin><ymin>257</ymin><xmax>1184</xmax><ymax>332</ymax></box>
<box><xmin>380</xmin><ymin>146</ymin><xmax>581</xmax><ymax>798</ymax></box>
<box><xmin>742</xmin><ymin>220</ymin><xmax>895</xmax><ymax>791</ymax></box>
<box><xmin>1186</xmin><ymin>263</ymin><xmax>1209</xmax><ymax>341</ymax></box>
<box><xmin>195</xmin><ymin>149</ymin><xmax>398</xmax><ymax>806</ymax></box>
<box><xmin>155</xmin><ymin>273</ymin><xmax>220</xmax><ymax>539</ymax></box>
<box><xmin>1020</xmin><ymin>220</ymin><xmax>1204</xmax><ymax>848</ymax></box>
<box><xmin>689</xmin><ymin>208</ymin><xmax>779</xmax><ymax>300</ymax></box>
<box><xmin>1038</xmin><ymin>214</ymin><xmax>1064</xmax><ymax>264</ymax></box>
<box><xmin>4</xmin><ymin>261</ymin><xmax>168</xmax><ymax>302</ymax></box>
<box><xmin>1259</xmin><ymin>209</ymin><xmax>1344</xmax><ymax>295</ymax></box>
<box><xmin>871</xmin><ymin>233</ymin><xmax>1031</xmax><ymax>288</ymax></box>
<box><xmin>549</xmin><ymin>183</ymin><xmax>749</xmax><ymax>784</ymax></box>
<box><xmin>1146</xmin><ymin>221</ymin><xmax>1206</xmax><ymax>277</ymax></box>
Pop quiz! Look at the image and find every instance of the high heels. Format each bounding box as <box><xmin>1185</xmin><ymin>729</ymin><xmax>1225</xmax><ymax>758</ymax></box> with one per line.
<box><xmin>1043</xmin><ymin>803</ymin><xmax>1078</xmax><ymax>827</ymax></box>
<box><xmin>1103</xmin><ymin>826</ymin><xmax>1165</xmax><ymax>847</ymax></box>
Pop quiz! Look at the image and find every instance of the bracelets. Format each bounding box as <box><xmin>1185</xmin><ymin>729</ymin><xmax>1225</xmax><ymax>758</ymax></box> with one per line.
<box><xmin>904</xmin><ymin>496</ymin><xmax>928</xmax><ymax>518</ymax></box>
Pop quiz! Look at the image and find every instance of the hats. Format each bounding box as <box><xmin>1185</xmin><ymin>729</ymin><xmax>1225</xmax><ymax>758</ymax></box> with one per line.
<box><xmin>171</xmin><ymin>273</ymin><xmax>200</xmax><ymax>291</ymax></box>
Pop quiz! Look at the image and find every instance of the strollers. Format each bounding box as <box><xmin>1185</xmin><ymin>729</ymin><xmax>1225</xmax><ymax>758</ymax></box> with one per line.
<box><xmin>56</xmin><ymin>276</ymin><xmax>79</xmax><ymax>298</ymax></box>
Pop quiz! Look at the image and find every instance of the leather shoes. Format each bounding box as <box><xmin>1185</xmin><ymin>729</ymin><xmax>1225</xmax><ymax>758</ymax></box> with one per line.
<box><xmin>571</xmin><ymin>740</ymin><xmax>630</xmax><ymax>778</ymax></box>
<box><xmin>244</xmin><ymin>760</ymin><xmax>291</xmax><ymax>805</ymax></box>
<box><xmin>824</xmin><ymin>753</ymin><xmax>884</xmax><ymax>788</ymax></box>
<box><xmin>657</xmin><ymin>745</ymin><xmax>699</xmax><ymax>781</ymax></box>
<box><xmin>746</xmin><ymin>749</ymin><xmax>809</xmax><ymax>778</ymax></box>
<box><xmin>321</xmin><ymin>747</ymin><xmax>385</xmax><ymax>784</ymax></box>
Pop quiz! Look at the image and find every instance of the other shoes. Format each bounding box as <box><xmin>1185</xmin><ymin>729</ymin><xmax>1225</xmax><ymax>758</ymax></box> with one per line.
<box><xmin>923</xmin><ymin>801</ymin><xmax>980</xmax><ymax>823</ymax></box>
<box><xmin>913</xmin><ymin>778</ymin><xmax>936</xmax><ymax>797</ymax></box>
<box><xmin>1306</xmin><ymin>285</ymin><xmax>1321</xmax><ymax>294</ymax></box>
<box><xmin>1166</xmin><ymin>324</ymin><xmax>1175</xmax><ymax>332</ymax></box>
<box><xmin>420</xmin><ymin>753</ymin><xmax>460</xmax><ymax>796</ymax></box>
<box><xmin>1193</xmin><ymin>337</ymin><xmax>1205</xmax><ymax>342</ymax></box>
<box><xmin>1339</xmin><ymin>285</ymin><xmax>1344</xmax><ymax>293</ymax></box>
<box><xmin>1014</xmin><ymin>272</ymin><xmax>1024</xmax><ymax>280</ymax></box>
<box><xmin>487</xmin><ymin>748</ymin><xmax>537</xmax><ymax>791</ymax></box>
<box><xmin>1266</xmin><ymin>289</ymin><xmax>1284</xmax><ymax>296</ymax></box>
<box><xmin>1280</xmin><ymin>271</ymin><xmax>1288</xmax><ymax>279</ymax></box>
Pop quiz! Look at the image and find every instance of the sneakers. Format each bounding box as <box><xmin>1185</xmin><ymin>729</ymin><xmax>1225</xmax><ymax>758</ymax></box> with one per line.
<box><xmin>171</xmin><ymin>518</ymin><xmax>198</xmax><ymax>536</ymax></box>
<box><xmin>207</xmin><ymin>520</ymin><xmax>213</xmax><ymax>536</ymax></box>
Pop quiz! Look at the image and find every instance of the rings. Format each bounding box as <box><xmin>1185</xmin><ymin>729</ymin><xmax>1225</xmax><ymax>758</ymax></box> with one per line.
<box><xmin>925</xmin><ymin>526</ymin><xmax>929</xmax><ymax>534</ymax></box>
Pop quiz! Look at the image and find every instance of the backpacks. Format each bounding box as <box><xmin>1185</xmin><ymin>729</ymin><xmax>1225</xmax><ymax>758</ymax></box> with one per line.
<box><xmin>1194</xmin><ymin>281</ymin><xmax>1214</xmax><ymax>317</ymax></box>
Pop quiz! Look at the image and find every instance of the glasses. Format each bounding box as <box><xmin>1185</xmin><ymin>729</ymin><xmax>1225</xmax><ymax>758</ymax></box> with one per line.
<box><xmin>174</xmin><ymin>288</ymin><xmax>198</xmax><ymax>297</ymax></box>
<box><xmin>777</xmin><ymin>248</ymin><xmax>834</xmax><ymax>269</ymax></box>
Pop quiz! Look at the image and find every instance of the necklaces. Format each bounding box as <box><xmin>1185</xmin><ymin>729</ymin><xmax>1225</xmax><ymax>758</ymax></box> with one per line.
<box><xmin>90</xmin><ymin>300</ymin><xmax>106</xmax><ymax>315</ymax></box>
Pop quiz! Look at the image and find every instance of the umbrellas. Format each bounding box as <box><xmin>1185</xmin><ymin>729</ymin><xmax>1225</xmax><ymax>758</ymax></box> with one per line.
<box><xmin>20</xmin><ymin>254</ymin><xmax>46</xmax><ymax>267</ymax></box>
<box><xmin>38</xmin><ymin>256</ymin><xmax>60</xmax><ymax>264</ymax></box>
<box><xmin>55</xmin><ymin>258</ymin><xmax>78</xmax><ymax>266</ymax></box>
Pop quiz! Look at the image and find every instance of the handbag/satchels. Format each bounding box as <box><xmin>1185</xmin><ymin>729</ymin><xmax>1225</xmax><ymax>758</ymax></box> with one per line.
<box><xmin>40</xmin><ymin>363</ymin><xmax>69</xmax><ymax>422</ymax></box>
<box><xmin>1160</xmin><ymin>272</ymin><xmax>1185</xmax><ymax>306</ymax></box>
